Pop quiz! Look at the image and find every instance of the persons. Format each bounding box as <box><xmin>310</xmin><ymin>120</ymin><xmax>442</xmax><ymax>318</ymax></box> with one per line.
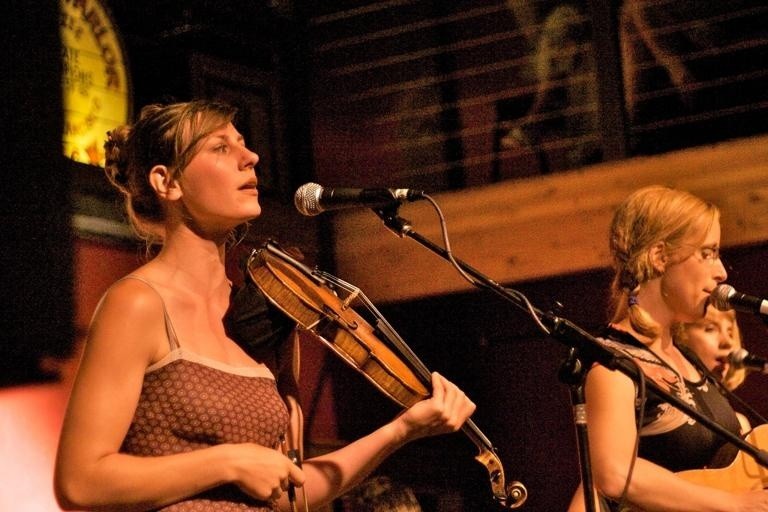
<box><xmin>500</xmin><ymin>4</ymin><xmax>695</xmax><ymax>166</ymax></box>
<box><xmin>335</xmin><ymin>471</ymin><xmax>420</xmax><ymax>512</ymax></box>
<box><xmin>584</xmin><ymin>184</ymin><xmax>768</xmax><ymax>512</ymax></box>
<box><xmin>59</xmin><ymin>98</ymin><xmax>479</xmax><ymax>511</ymax></box>
<box><xmin>566</xmin><ymin>301</ymin><xmax>752</xmax><ymax>512</ymax></box>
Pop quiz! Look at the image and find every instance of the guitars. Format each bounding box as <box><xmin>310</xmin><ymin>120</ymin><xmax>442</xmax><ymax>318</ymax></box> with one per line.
<box><xmin>568</xmin><ymin>421</ymin><xmax>767</xmax><ymax>512</ymax></box>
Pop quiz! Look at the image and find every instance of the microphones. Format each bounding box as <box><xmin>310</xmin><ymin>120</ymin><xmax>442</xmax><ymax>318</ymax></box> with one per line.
<box><xmin>709</xmin><ymin>283</ymin><xmax>768</xmax><ymax>315</ymax></box>
<box><xmin>727</xmin><ymin>348</ymin><xmax>768</xmax><ymax>374</ymax></box>
<box><xmin>293</xmin><ymin>182</ymin><xmax>426</xmax><ymax>217</ymax></box>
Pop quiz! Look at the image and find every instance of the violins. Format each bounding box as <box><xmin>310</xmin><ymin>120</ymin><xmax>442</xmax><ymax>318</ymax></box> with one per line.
<box><xmin>246</xmin><ymin>235</ymin><xmax>529</xmax><ymax>508</ymax></box>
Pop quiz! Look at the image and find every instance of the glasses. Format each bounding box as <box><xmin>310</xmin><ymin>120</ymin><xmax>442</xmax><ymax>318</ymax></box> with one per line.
<box><xmin>667</xmin><ymin>237</ymin><xmax>721</xmax><ymax>260</ymax></box>
